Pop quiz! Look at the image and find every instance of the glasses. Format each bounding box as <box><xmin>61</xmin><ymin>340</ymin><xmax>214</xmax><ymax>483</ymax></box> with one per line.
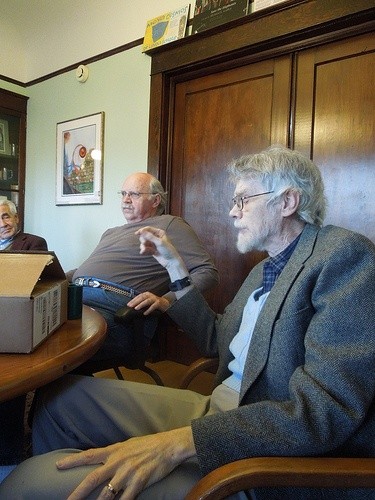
<box><xmin>232</xmin><ymin>191</ymin><xmax>275</xmax><ymax>211</ymax></box>
<box><xmin>116</xmin><ymin>191</ymin><xmax>156</xmax><ymax>199</ymax></box>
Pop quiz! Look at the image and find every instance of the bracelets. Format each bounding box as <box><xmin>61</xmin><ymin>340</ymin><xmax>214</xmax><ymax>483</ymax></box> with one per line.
<box><xmin>168</xmin><ymin>276</ymin><xmax>193</xmax><ymax>292</ymax></box>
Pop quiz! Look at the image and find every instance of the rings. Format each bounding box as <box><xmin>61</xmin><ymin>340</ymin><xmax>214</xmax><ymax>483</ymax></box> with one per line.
<box><xmin>106</xmin><ymin>482</ymin><xmax>118</xmax><ymax>495</ymax></box>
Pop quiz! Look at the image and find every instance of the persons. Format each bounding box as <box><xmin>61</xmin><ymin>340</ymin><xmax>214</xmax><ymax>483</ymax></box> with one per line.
<box><xmin>66</xmin><ymin>171</ymin><xmax>217</xmax><ymax>377</ymax></box>
<box><xmin>0</xmin><ymin>197</ymin><xmax>49</xmax><ymax>253</ymax></box>
<box><xmin>0</xmin><ymin>145</ymin><xmax>375</xmax><ymax>500</ymax></box>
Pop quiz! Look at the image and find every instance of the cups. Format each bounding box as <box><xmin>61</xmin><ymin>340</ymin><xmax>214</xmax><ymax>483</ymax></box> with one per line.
<box><xmin>0</xmin><ymin>167</ymin><xmax>13</xmax><ymax>181</ymax></box>
<box><xmin>67</xmin><ymin>284</ymin><xmax>82</xmax><ymax>320</ymax></box>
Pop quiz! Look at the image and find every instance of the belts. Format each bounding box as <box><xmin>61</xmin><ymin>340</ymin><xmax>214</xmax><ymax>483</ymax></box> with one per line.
<box><xmin>77</xmin><ymin>280</ymin><xmax>137</xmax><ymax>300</ymax></box>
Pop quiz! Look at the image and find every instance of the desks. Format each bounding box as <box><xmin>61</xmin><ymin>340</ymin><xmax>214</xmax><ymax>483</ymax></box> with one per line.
<box><xmin>0</xmin><ymin>301</ymin><xmax>104</xmax><ymax>400</ymax></box>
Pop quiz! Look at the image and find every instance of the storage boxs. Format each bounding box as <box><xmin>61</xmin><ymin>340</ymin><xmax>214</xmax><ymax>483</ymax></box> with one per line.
<box><xmin>0</xmin><ymin>250</ymin><xmax>69</xmax><ymax>354</ymax></box>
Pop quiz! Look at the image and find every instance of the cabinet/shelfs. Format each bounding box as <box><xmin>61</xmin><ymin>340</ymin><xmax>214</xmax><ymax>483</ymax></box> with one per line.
<box><xmin>0</xmin><ymin>88</ymin><xmax>31</xmax><ymax>233</ymax></box>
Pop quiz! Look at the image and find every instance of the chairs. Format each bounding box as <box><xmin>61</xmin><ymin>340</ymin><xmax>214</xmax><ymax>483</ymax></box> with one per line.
<box><xmin>77</xmin><ymin>280</ymin><xmax>375</xmax><ymax>500</ymax></box>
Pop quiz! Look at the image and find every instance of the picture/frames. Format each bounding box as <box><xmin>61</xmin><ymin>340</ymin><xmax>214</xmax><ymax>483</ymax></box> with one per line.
<box><xmin>56</xmin><ymin>111</ymin><xmax>105</xmax><ymax>205</ymax></box>
